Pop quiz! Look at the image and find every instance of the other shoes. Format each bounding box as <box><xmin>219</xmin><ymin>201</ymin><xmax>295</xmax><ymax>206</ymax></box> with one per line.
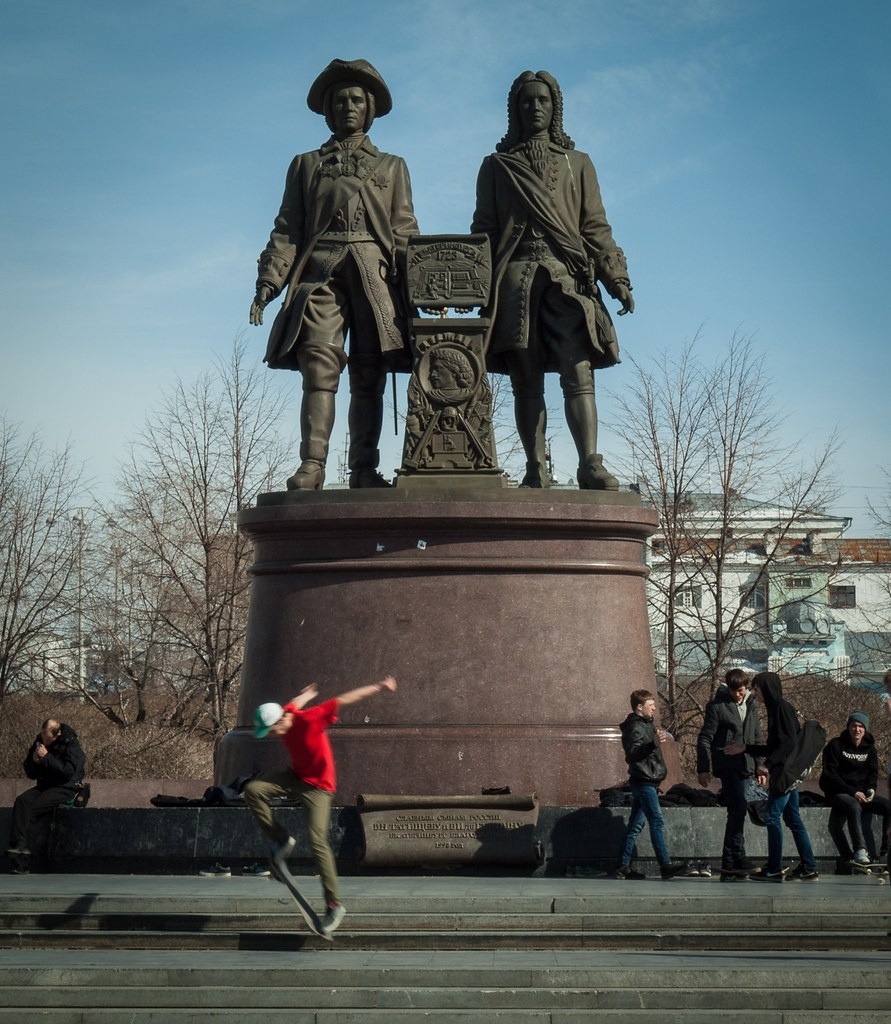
<box><xmin>615</xmin><ymin>868</ymin><xmax>645</xmax><ymax>880</ymax></box>
<box><xmin>279</xmin><ymin>837</ymin><xmax>295</xmax><ymax>859</ymax></box>
<box><xmin>661</xmin><ymin>862</ymin><xmax>690</xmax><ymax>879</ymax></box>
<box><xmin>321</xmin><ymin>905</ymin><xmax>344</xmax><ymax>932</ymax></box>
<box><xmin>680</xmin><ymin>860</ymin><xmax>699</xmax><ymax>876</ymax></box>
<box><xmin>721</xmin><ymin>868</ymin><xmax>743</xmax><ymax>877</ymax></box>
<box><xmin>7</xmin><ymin>848</ymin><xmax>31</xmax><ymax>859</ymax></box>
<box><xmin>700</xmin><ymin>861</ymin><xmax>713</xmax><ymax>877</ymax></box>
<box><xmin>735</xmin><ymin>863</ymin><xmax>759</xmax><ymax>872</ymax></box>
<box><xmin>12</xmin><ymin>865</ymin><xmax>30</xmax><ymax>874</ymax></box>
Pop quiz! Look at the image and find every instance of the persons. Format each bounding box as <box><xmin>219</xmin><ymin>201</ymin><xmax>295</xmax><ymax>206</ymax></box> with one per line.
<box><xmin>820</xmin><ymin>710</ymin><xmax>891</xmax><ymax>869</ymax></box>
<box><xmin>7</xmin><ymin>718</ymin><xmax>87</xmax><ymax>856</ymax></box>
<box><xmin>875</xmin><ymin>669</ymin><xmax>891</xmax><ymax>883</ymax></box>
<box><xmin>243</xmin><ymin>676</ymin><xmax>399</xmax><ymax>936</ymax></box>
<box><xmin>695</xmin><ymin>669</ymin><xmax>818</xmax><ymax>882</ymax></box>
<box><xmin>619</xmin><ymin>689</ymin><xmax>693</xmax><ymax>879</ymax></box>
<box><xmin>470</xmin><ymin>69</ymin><xmax>636</xmax><ymax>493</ymax></box>
<box><xmin>249</xmin><ymin>57</ymin><xmax>423</xmax><ymax>493</ymax></box>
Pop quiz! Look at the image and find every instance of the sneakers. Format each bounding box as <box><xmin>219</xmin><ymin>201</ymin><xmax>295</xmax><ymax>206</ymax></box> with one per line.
<box><xmin>856</xmin><ymin>848</ymin><xmax>870</xmax><ymax>864</ymax></box>
<box><xmin>785</xmin><ymin>863</ymin><xmax>819</xmax><ymax>881</ymax></box>
<box><xmin>242</xmin><ymin>863</ymin><xmax>271</xmax><ymax>877</ymax></box>
<box><xmin>750</xmin><ymin>870</ymin><xmax>782</xmax><ymax>882</ymax></box>
<box><xmin>199</xmin><ymin>863</ymin><xmax>231</xmax><ymax>877</ymax></box>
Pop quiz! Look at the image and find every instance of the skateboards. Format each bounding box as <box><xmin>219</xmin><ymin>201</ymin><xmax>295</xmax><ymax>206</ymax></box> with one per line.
<box><xmin>843</xmin><ymin>858</ymin><xmax>890</xmax><ymax>886</ymax></box>
<box><xmin>718</xmin><ymin>866</ymin><xmax>790</xmax><ymax>882</ymax></box>
<box><xmin>260</xmin><ymin>845</ymin><xmax>334</xmax><ymax>942</ymax></box>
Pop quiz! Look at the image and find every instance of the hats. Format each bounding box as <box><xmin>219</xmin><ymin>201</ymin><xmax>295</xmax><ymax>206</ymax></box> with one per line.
<box><xmin>252</xmin><ymin>702</ymin><xmax>285</xmax><ymax>739</ymax></box>
<box><xmin>847</xmin><ymin>710</ymin><xmax>870</xmax><ymax>732</ymax></box>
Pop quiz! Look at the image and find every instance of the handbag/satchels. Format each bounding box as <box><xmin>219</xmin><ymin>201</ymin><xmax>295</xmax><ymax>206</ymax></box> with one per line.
<box><xmin>773</xmin><ymin>719</ymin><xmax>826</xmax><ymax>795</ymax></box>
